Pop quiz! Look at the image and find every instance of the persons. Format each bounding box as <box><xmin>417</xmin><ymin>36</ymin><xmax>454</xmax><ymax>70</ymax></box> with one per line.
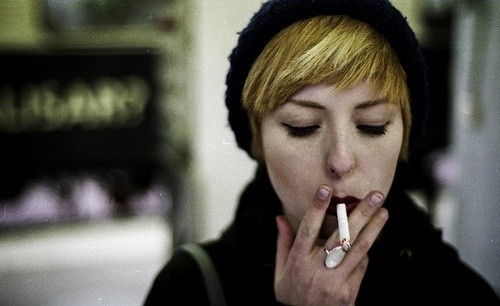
<box><xmin>143</xmin><ymin>0</ymin><xmax>500</xmax><ymax>306</ymax></box>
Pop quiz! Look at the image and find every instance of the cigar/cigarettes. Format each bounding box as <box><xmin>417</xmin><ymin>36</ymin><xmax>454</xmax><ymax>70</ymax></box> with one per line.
<box><xmin>335</xmin><ymin>203</ymin><xmax>352</xmax><ymax>253</ymax></box>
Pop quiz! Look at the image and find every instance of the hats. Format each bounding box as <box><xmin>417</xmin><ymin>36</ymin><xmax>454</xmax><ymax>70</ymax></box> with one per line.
<box><xmin>225</xmin><ymin>0</ymin><xmax>440</xmax><ymax>162</ymax></box>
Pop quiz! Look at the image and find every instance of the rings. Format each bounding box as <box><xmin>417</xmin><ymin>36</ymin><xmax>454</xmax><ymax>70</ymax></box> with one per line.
<box><xmin>324</xmin><ymin>246</ymin><xmax>345</xmax><ymax>269</ymax></box>
